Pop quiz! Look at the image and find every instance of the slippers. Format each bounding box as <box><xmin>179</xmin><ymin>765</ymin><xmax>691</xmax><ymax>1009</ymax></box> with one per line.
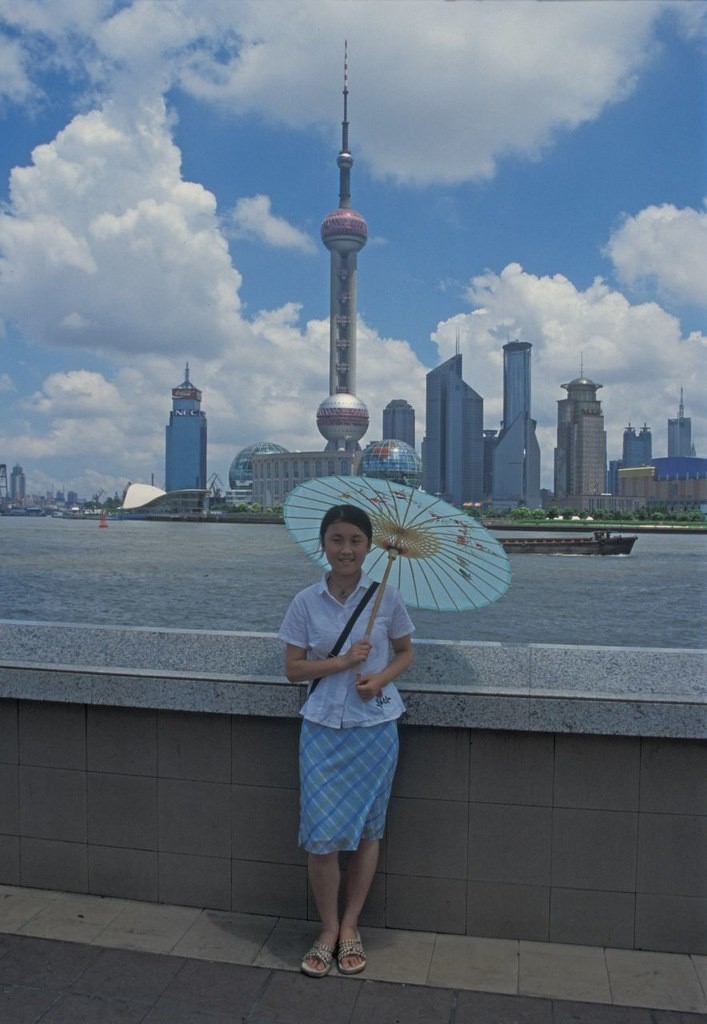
<box><xmin>301</xmin><ymin>940</ymin><xmax>337</xmax><ymax>978</ymax></box>
<box><xmin>338</xmin><ymin>930</ymin><xmax>367</xmax><ymax>974</ymax></box>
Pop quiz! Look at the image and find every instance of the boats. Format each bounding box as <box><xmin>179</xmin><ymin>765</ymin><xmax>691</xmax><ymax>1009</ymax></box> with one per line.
<box><xmin>496</xmin><ymin>528</ymin><xmax>639</xmax><ymax>554</ymax></box>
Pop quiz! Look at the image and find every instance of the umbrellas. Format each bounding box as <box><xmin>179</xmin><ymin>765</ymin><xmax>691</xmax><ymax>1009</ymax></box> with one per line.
<box><xmin>283</xmin><ymin>474</ymin><xmax>513</xmax><ymax>674</ymax></box>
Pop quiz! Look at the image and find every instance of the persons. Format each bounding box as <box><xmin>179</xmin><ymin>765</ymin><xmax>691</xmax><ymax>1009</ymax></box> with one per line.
<box><xmin>277</xmin><ymin>504</ymin><xmax>416</xmax><ymax>978</ymax></box>
